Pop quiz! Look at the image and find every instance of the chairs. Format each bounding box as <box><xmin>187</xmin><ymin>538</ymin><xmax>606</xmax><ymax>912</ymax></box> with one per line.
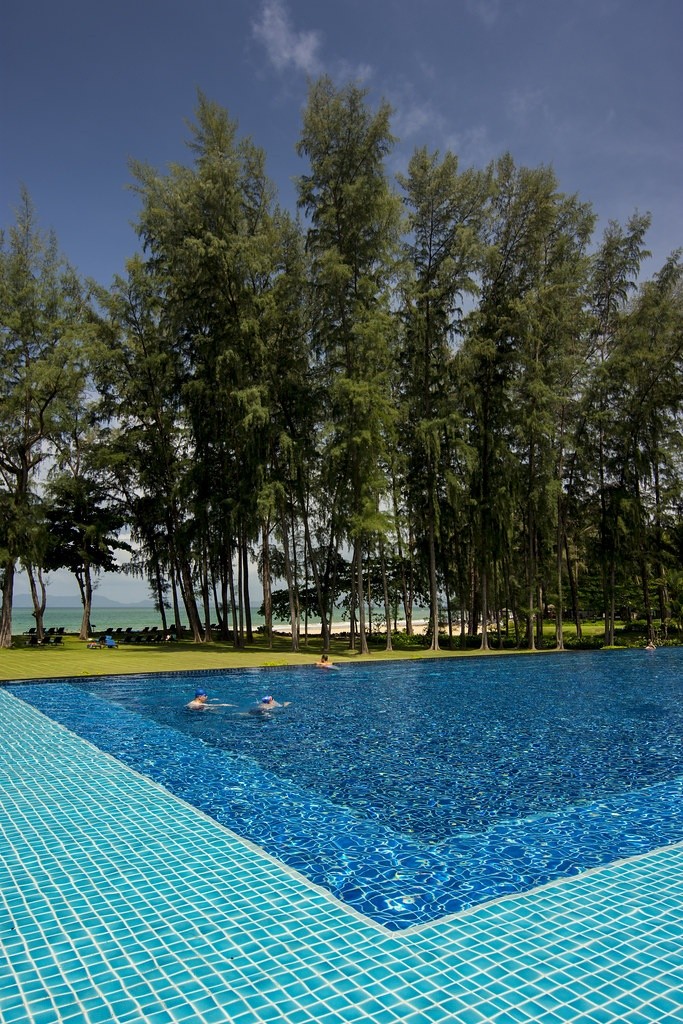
<box><xmin>203</xmin><ymin>621</ymin><xmax>222</xmax><ymax>631</ymax></box>
<box><xmin>88</xmin><ymin>624</ymin><xmax>182</xmax><ymax>649</ymax></box>
<box><xmin>22</xmin><ymin>626</ymin><xmax>67</xmax><ymax>647</ymax></box>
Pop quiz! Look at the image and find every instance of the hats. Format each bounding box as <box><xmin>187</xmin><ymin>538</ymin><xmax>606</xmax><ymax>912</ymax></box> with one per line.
<box><xmin>195</xmin><ymin>689</ymin><xmax>206</xmax><ymax>696</ymax></box>
<box><xmin>262</xmin><ymin>696</ymin><xmax>270</xmax><ymax>704</ymax></box>
<box><xmin>322</xmin><ymin>654</ymin><xmax>328</xmax><ymax>661</ymax></box>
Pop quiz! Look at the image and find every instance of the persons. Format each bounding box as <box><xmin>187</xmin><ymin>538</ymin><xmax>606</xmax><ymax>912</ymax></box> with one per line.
<box><xmin>191</xmin><ymin>688</ymin><xmax>207</xmax><ymax>705</ymax></box>
<box><xmin>646</xmin><ymin>639</ymin><xmax>656</xmax><ymax>649</ymax></box>
<box><xmin>316</xmin><ymin>654</ymin><xmax>333</xmax><ymax>666</ymax></box>
<box><xmin>261</xmin><ymin>696</ymin><xmax>274</xmax><ymax>704</ymax></box>
<box><xmin>98</xmin><ymin>632</ymin><xmax>106</xmax><ymax>642</ymax></box>
